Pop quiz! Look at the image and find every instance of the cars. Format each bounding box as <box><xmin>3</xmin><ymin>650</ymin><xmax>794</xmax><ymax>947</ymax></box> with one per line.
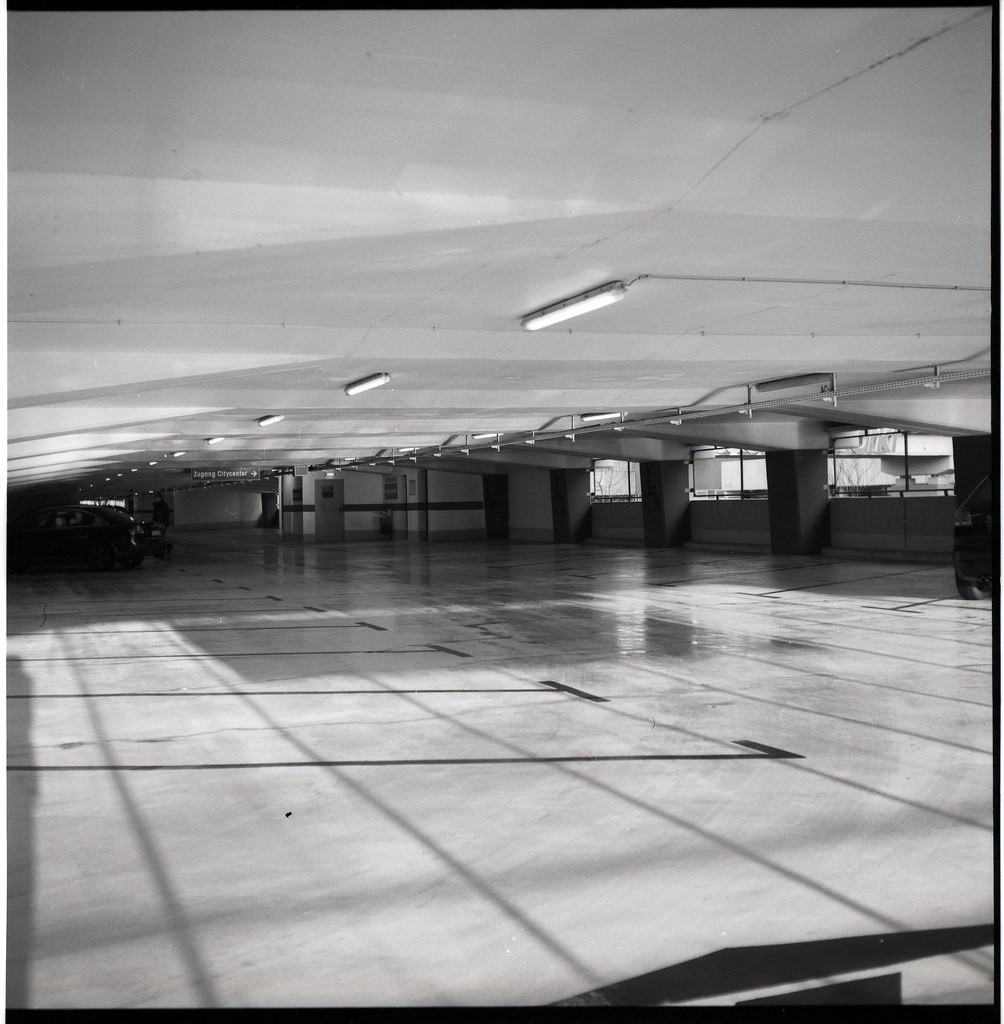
<box><xmin>7</xmin><ymin>505</ymin><xmax>167</xmax><ymax>572</ymax></box>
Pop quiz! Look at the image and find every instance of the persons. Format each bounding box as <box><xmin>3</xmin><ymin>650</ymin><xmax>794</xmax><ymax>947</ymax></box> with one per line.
<box><xmin>151</xmin><ymin>492</ymin><xmax>174</xmax><ymax>552</ymax></box>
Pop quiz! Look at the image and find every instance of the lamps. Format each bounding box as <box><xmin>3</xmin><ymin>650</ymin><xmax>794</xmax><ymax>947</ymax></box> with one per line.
<box><xmin>170</xmin><ymin>280</ymin><xmax>627</xmax><ymax>460</ymax></box>
